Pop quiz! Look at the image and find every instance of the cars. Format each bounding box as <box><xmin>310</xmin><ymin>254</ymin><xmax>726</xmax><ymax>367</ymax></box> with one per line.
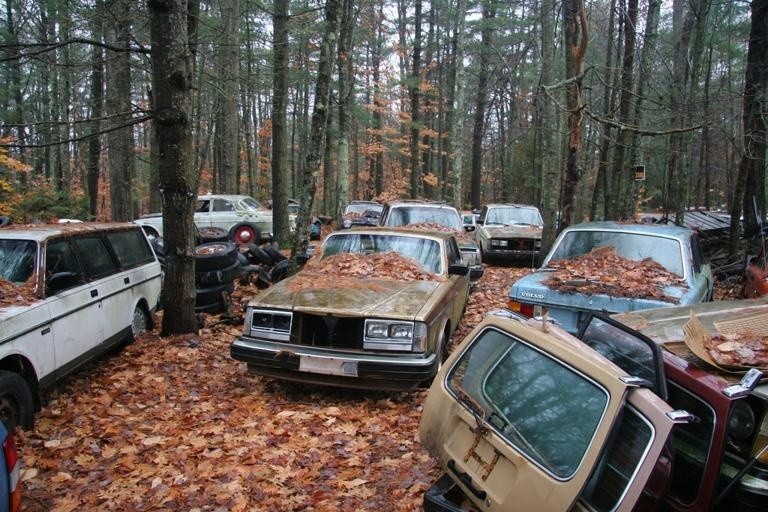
<box><xmin>342</xmin><ymin>201</ymin><xmax>384</xmax><ymax>228</ymax></box>
<box><xmin>378</xmin><ymin>200</ymin><xmax>485</xmax><ymax>284</ymax></box>
<box><xmin>657</xmin><ymin>210</ymin><xmax>767</xmax><ymax>281</ymax></box>
<box><xmin>227</xmin><ymin>226</ymin><xmax>472</xmax><ymax>402</ymax></box>
<box><xmin>473</xmin><ymin>203</ymin><xmax>543</xmax><ymax>267</ymax></box>
<box><xmin>268</xmin><ymin>200</ymin><xmax>322</xmax><ymax>240</ymax></box>
<box><xmin>141</xmin><ymin>194</ymin><xmax>299</xmax><ymax>246</ymax></box>
<box><xmin>504</xmin><ymin>216</ymin><xmax>711</xmax><ymax>342</ymax></box>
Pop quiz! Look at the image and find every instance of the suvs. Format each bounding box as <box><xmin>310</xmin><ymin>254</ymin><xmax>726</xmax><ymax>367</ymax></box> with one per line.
<box><xmin>0</xmin><ymin>217</ymin><xmax>166</xmax><ymax>433</ymax></box>
<box><xmin>582</xmin><ymin>288</ymin><xmax>768</xmax><ymax>508</ymax></box>
